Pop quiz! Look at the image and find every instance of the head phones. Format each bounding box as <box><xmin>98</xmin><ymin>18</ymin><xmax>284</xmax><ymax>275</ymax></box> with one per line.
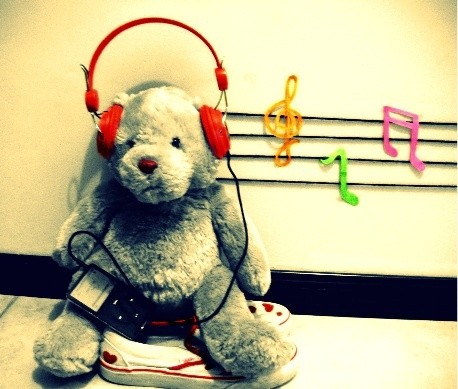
<box><xmin>79</xmin><ymin>18</ymin><xmax>231</xmax><ymax>160</ymax></box>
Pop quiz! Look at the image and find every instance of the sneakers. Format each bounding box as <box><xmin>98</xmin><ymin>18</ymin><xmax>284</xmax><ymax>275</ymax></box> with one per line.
<box><xmin>127</xmin><ymin>293</ymin><xmax>292</xmax><ymax>332</ymax></box>
<box><xmin>99</xmin><ymin>328</ymin><xmax>302</xmax><ymax>389</ymax></box>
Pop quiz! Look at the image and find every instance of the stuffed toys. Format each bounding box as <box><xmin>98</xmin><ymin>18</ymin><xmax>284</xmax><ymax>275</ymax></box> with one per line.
<box><xmin>32</xmin><ymin>87</ymin><xmax>294</xmax><ymax>378</ymax></box>
